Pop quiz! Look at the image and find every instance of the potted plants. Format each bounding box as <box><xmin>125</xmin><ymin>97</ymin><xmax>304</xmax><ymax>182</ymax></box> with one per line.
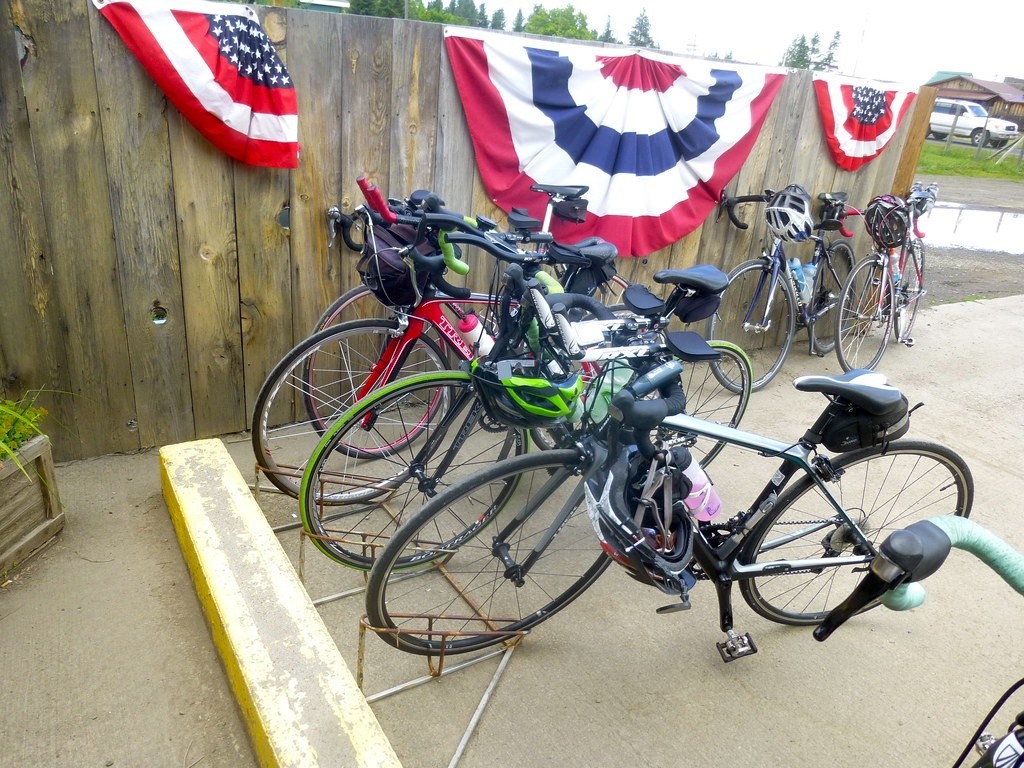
<box><xmin>0</xmin><ymin>386</ymin><xmax>66</xmax><ymax>580</ymax></box>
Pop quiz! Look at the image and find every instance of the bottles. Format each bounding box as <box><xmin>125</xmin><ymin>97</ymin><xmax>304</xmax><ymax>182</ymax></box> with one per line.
<box><xmin>458</xmin><ymin>313</ymin><xmax>495</xmax><ymax>357</ymax></box>
<box><xmin>585</xmin><ymin>345</ymin><xmax>637</xmax><ymax>424</ymax></box>
<box><xmin>544</xmin><ymin>358</ymin><xmax>585</xmax><ymax>424</ymax></box>
<box><xmin>787</xmin><ymin>257</ymin><xmax>811</xmax><ymax>304</ymax></box>
<box><xmin>668</xmin><ymin>446</ymin><xmax>722</xmax><ymax>522</ymax></box>
<box><xmin>889</xmin><ymin>248</ymin><xmax>900</xmax><ymax>282</ymax></box>
<box><xmin>803</xmin><ymin>262</ymin><xmax>815</xmax><ymax>293</ymax></box>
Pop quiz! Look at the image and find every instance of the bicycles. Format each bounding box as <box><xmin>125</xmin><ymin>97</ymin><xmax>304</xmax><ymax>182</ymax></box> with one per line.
<box><xmin>704</xmin><ymin>183</ymin><xmax>859</xmax><ymax>394</ymax></box>
<box><xmin>815</xmin><ymin>516</ymin><xmax>1024</xmax><ymax>768</ymax></box>
<box><xmin>364</xmin><ymin>265</ymin><xmax>973</xmax><ymax>664</ymax></box>
<box><xmin>836</xmin><ymin>181</ymin><xmax>939</xmax><ymax>376</ymax></box>
<box><xmin>252</xmin><ymin>173</ymin><xmax>752</xmax><ymax>572</ymax></box>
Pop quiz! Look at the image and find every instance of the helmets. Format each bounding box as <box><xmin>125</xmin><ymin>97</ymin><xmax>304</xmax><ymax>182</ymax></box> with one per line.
<box><xmin>472</xmin><ymin>353</ymin><xmax>583</xmax><ymax>428</ymax></box>
<box><xmin>864</xmin><ymin>195</ymin><xmax>910</xmax><ymax>249</ymax></box>
<box><xmin>583</xmin><ymin>456</ymin><xmax>698</xmax><ymax>596</ymax></box>
<box><xmin>764</xmin><ymin>184</ymin><xmax>814</xmax><ymax>244</ymax></box>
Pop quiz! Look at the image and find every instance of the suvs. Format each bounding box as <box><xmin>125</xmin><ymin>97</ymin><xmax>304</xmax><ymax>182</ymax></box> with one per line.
<box><xmin>925</xmin><ymin>99</ymin><xmax>1019</xmax><ymax>147</ymax></box>
